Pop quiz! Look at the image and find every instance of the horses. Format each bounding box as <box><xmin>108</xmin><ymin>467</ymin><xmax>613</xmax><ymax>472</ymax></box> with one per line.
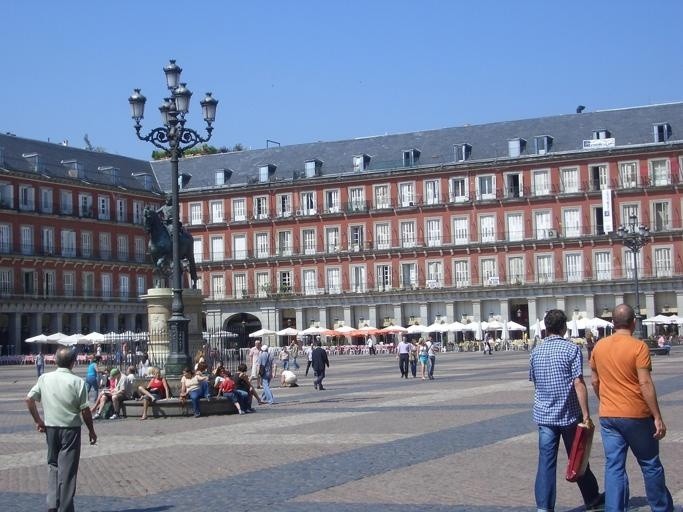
<box><xmin>142</xmin><ymin>206</ymin><xmax>198</xmax><ymax>289</ymax></box>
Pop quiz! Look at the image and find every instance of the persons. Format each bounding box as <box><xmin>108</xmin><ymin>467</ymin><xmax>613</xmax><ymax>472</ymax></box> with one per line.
<box><xmin>24</xmin><ymin>347</ymin><xmax>97</xmax><ymax>510</ymax></box>
<box><xmin>35</xmin><ymin>352</ymin><xmax>44</xmax><ymax>377</ymax></box>
<box><xmin>649</xmin><ymin>332</ymin><xmax>678</xmax><ymax>348</ymax></box>
<box><xmin>584</xmin><ymin>332</ymin><xmax>602</xmax><ymax>359</ymax></box>
<box><xmin>527</xmin><ymin>309</ymin><xmax>606</xmax><ymax>512</ymax></box>
<box><xmin>153</xmin><ymin>194</ymin><xmax>181</xmax><ymax>245</ymax></box>
<box><xmin>397</xmin><ymin>336</ymin><xmax>438</xmax><ymax>380</ymax></box>
<box><xmin>181</xmin><ymin>339</ymin><xmax>275</xmax><ymax>418</ymax></box>
<box><xmin>483</xmin><ymin>331</ymin><xmax>493</xmax><ymax>355</ymax></box>
<box><xmin>588</xmin><ymin>304</ymin><xmax>673</xmax><ymax>512</ymax></box>
<box><xmin>86</xmin><ymin>344</ymin><xmax>168</xmax><ymax>420</ymax></box>
<box><xmin>278</xmin><ymin>336</ymin><xmax>330</xmax><ymax>391</ymax></box>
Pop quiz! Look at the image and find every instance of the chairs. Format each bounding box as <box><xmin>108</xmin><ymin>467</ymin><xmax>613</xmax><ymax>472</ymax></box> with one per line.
<box><xmin>221</xmin><ymin>339</ymin><xmax>529</xmax><ymax>366</ymax></box>
<box><xmin>0</xmin><ymin>338</ymin><xmax>147</xmax><ymax>366</ymax></box>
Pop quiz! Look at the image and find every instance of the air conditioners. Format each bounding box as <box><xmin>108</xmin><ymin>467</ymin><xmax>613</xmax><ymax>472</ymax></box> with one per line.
<box><xmin>296</xmin><ymin>210</ymin><xmax>303</xmax><ymax>216</ymax></box>
<box><xmin>408</xmin><ymin>201</ymin><xmax>417</xmax><ymax>206</ymax></box>
<box><xmin>547</xmin><ymin>230</ymin><xmax>558</xmax><ymax>238</ymax></box>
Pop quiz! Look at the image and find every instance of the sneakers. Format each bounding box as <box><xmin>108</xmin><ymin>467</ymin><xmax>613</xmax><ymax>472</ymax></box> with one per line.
<box><xmin>92</xmin><ymin>414</ymin><xmax>102</xmax><ymax>420</ymax></box>
<box><xmin>109</xmin><ymin>414</ymin><xmax>119</xmax><ymax>420</ymax></box>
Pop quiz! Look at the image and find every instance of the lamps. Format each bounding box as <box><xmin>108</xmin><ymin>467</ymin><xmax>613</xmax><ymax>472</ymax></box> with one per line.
<box><xmin>287</xmin><ymin>301</ymin><xmax>670</xmax><ymax>328</ymax></box>
<box><xmin>241</xmin><ymin>319</ymin><xmax>246</xmax><ymax>327</ymax></box>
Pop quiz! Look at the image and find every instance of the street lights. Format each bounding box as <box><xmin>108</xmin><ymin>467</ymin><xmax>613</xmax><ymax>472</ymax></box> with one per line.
<box><xmin>127</xmin><ymin>59</ymin><xmax>218</xmax><ymax>375</ymax></box>
<box><xmin>617</xmin><ymin>211</ymin><xmax>650</xmax><ymax>339</ymax></box>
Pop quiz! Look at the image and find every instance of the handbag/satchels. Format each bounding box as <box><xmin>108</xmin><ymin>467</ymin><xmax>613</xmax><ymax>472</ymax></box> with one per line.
<box><xmin>565</xmin><ymin>424</ymin><xmax>594</xmax><ymax>482</ymax></box>
<box><xmin>259</xmin><ymin>365</ymin><xmax>265</xmax><ymax>377</ymax></box>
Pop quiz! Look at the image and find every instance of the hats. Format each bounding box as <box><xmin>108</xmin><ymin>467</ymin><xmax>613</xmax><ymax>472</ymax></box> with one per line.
<box><xmin>109</xmin><ymin>368</ymin><xmax>119</xmax><ymax>380</ymax></box>
